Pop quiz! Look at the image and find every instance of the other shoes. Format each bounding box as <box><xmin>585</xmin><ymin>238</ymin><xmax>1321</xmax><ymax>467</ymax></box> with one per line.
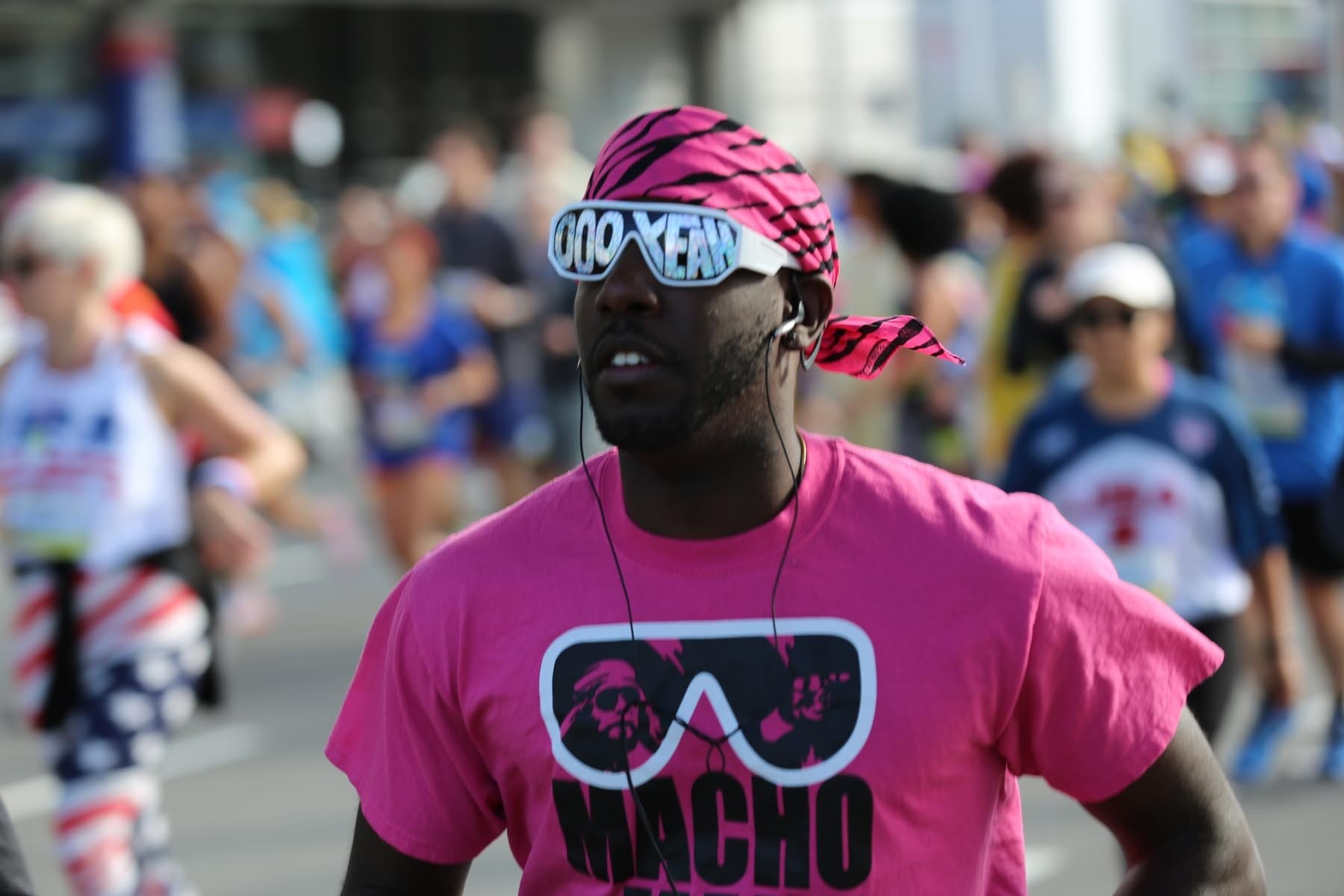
<box><xmin>1235</xmin><ymin>708</ymin><xmax>1296</xmax><ymax>782</ymax></box>
<box><xmin>1323</xmin><ymin>701</ymin><xmax>1344</xmax><ymax>782</ymax></box>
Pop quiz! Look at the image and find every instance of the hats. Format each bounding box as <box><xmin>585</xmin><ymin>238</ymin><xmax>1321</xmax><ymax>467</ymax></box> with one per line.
<box><xmin>1061</xmin><ymin>242</ymin><xmax>1176</xmax><ymax>310</ymax></box>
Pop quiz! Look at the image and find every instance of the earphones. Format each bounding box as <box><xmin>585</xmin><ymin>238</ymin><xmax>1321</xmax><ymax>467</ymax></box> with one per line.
<box><xmin>771</xmin><ymin>284</ymin><xmax>808</xmax><ymax>338</ymax></box>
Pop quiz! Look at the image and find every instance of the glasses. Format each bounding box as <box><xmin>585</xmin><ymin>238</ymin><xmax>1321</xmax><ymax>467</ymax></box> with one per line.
<box><xmin>547</xmin><ymin>200</ymin><xmax>802</xmax><ymax>287</ymax></box>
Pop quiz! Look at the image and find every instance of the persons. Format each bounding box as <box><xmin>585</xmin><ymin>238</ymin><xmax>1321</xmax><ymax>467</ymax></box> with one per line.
<box><xmin>816</xmin><ymin>79</ymin><xmax>1343</xmax><ymax>896</ymax></box>
<box><xmin>325</xmin><ymin>104</ymin><xmax>1271</xmax><ymax>896</ymax></box>
<box><xmin>0</xmin><ymin>106</ymin><xmax>864</xmax><ymax>894</ymax></box>
